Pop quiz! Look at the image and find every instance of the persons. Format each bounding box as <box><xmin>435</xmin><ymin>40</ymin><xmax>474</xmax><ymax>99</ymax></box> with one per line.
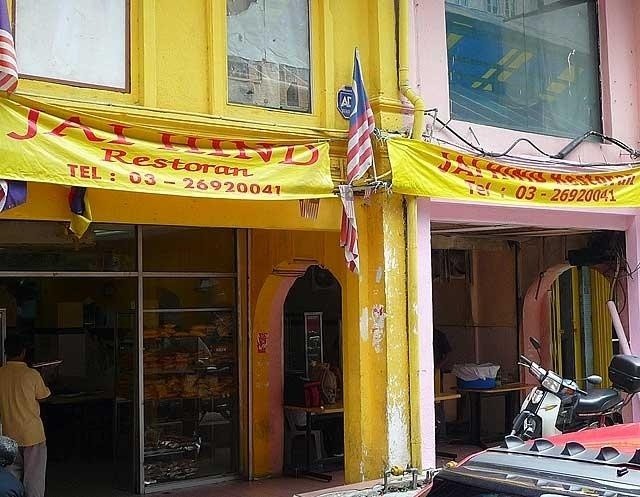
<box><xmin>434</xmin><ymin>325</ymin><xmax>452</xmax><ymax>442</ymax></box>
<box><xmin>0</xmin><ymin>332</ymin><xmax>52</xmax><ymax>496</ymax></box>
<box><xmin>0</xmin><ymin>435</ymin><xmax>24</xmax><ymax>497</ymax></box>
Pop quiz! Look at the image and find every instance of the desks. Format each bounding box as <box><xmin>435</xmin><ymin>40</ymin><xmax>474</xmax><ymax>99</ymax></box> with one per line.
<box><xmin>287</xmin><ymin>380</ymin><xmax>537</xmax><ymax>482</ymax></box>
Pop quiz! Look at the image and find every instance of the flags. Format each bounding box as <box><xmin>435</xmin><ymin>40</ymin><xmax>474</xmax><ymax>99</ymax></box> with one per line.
<box><xmin>346</xmin><ymin>46</ymin><xmax>375</xmax><ymax>186</ymax></box>
<box><xmin>0</xmin><ymin>0</ymin><xmax>19</xmax><ymax>95</ymax></box>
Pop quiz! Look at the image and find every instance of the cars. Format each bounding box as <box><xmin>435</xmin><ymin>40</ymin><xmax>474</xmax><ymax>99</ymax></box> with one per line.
<box><xmin>414</xmin><ymin>422</ymin><xmax>640</xmax><ymax>497</ymax></box>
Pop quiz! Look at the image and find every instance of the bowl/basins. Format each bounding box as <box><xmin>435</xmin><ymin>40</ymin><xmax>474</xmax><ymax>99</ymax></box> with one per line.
<box><xmin>455</xmin><ymin>365</ymin><xmax>500</xmax><ymax>390</ymax></box>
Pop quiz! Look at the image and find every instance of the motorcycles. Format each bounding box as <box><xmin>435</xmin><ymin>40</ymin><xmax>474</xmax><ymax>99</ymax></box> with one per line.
<box><xmin>509</xmin><ymin>336</ymin><xmax>640</xmax><ymax>441</ymax></box>
